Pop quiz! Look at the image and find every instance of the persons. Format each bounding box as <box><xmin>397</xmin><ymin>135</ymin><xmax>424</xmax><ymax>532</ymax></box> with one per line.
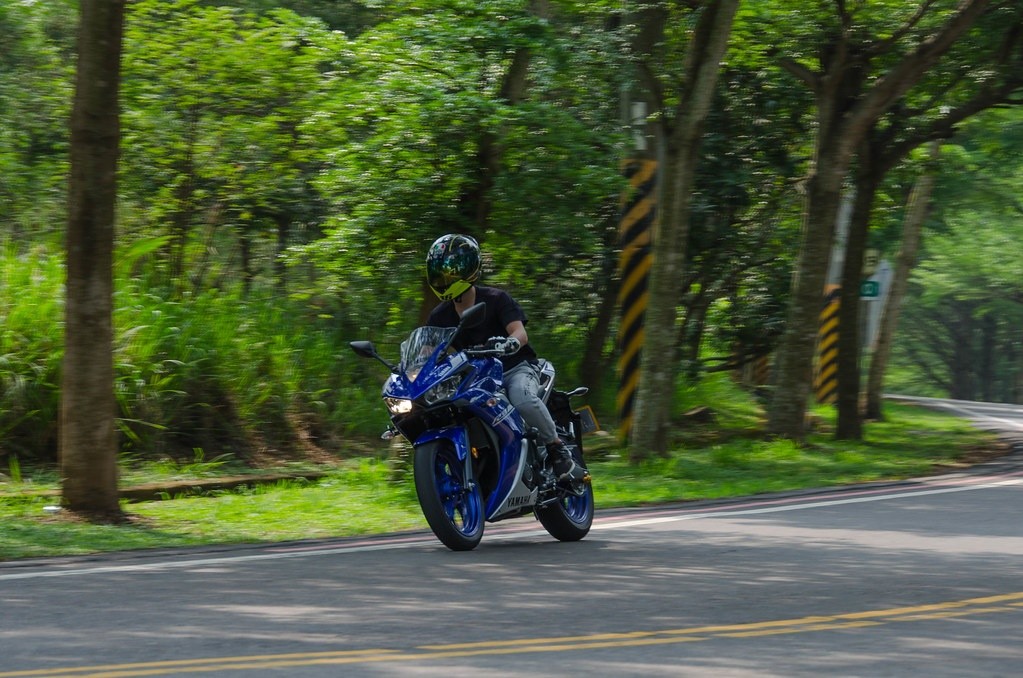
<box><xmin>418</xmin><ymin>233</ymin><xmax>576</xmax><ymax>482</ymax></box>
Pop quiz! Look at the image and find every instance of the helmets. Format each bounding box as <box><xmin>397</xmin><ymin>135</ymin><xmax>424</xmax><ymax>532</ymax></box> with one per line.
<box><xmin>425</xmin><ymin>233</ymin><xmax>482</xmax><ymax>303</ymax></box>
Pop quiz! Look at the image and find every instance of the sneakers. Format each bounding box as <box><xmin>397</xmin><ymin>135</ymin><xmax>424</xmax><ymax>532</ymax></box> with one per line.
<box><xmin>545</xmin><ymin>439</ymin><xmax>575</xmax><ymax>481</ymax></box>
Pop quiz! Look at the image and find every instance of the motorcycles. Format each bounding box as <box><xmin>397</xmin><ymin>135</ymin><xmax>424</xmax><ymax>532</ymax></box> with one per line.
<box><xmin>348</xmin><ymin>301</ymin><xmax>600</xmax><ymax>551</ymax></box>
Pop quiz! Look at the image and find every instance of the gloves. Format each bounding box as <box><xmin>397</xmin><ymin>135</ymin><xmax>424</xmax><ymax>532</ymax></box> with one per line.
<box><xmin>486</xmin><ymin>336</ymin><xmax>521</xmax><ymax>358</ymax></box>
